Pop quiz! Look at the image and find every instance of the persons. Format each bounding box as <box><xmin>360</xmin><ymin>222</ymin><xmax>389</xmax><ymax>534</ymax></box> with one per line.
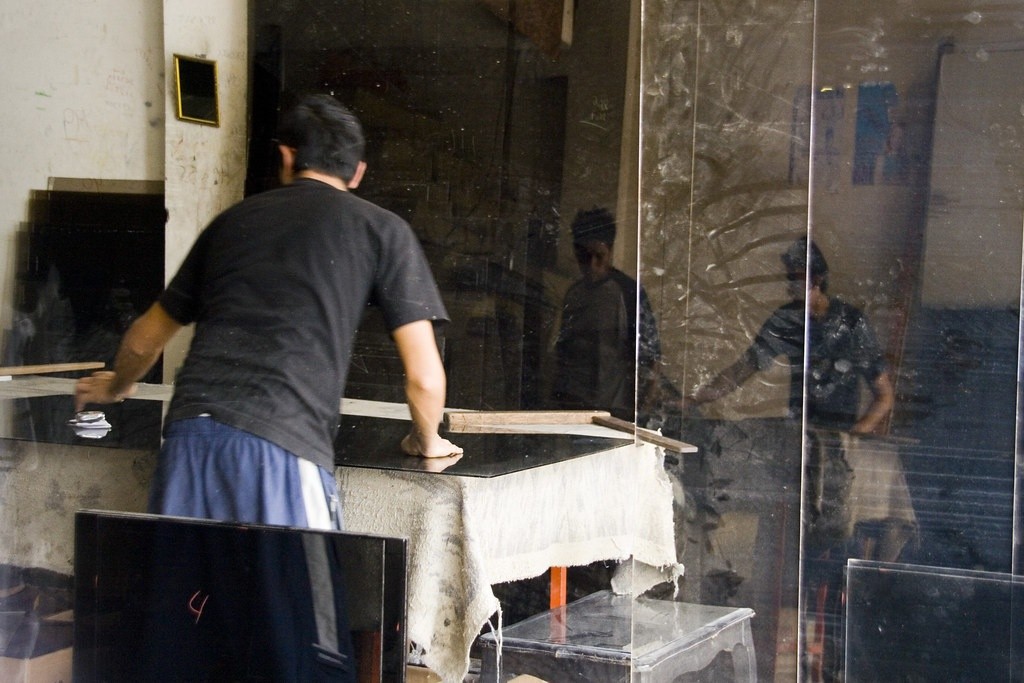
<box><xmin>692</xmin><ymin>235</ymin><xmax>895</xmax><ymax>433</ymax></box>
<box><xmin>547</xmin><ymin>208</ymin><xmax>661</xmax><ymax>421</ymax></box>
<box><xmin>74</xmin><ymin>96</ymin><xmax>463</xmax><ymax>534</ymax></box>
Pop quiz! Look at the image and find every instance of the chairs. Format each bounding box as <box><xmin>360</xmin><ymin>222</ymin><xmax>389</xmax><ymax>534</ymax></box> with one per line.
<box><xmin>72</xmin><ymin>507</ymin><xmax>411</xmax><ymax>683</ymax></box>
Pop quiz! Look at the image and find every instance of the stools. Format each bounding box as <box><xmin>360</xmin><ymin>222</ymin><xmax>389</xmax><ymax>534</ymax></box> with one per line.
<box><xmin>473</xmin><ymin>589</ymin><xmax>759</xmax><ymax>683</ymax></box>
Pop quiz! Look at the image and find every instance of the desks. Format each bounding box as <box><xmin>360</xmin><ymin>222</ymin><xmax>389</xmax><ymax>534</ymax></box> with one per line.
<box><xmin>0</xmin><ymin>376</ymin><xmax>685</xmax><ymax>683</ymax></box>
